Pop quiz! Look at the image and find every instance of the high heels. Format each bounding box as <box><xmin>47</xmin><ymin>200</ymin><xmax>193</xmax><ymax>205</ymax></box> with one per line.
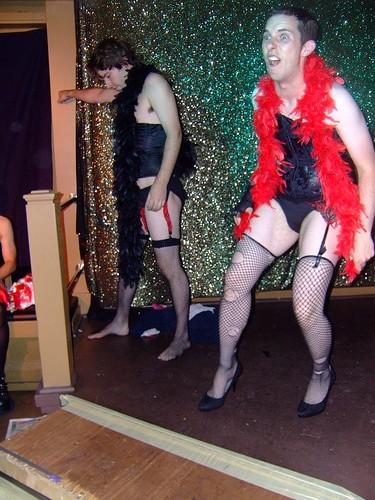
<box><xmin>198</xmin><ymin>357</ymin><xmax>242</xmax><ymax>412</ymax></box>
<box><xmin>299</xmin><ymin>366</ymin><xmax>337</xmax><ymax>417</ymax></box>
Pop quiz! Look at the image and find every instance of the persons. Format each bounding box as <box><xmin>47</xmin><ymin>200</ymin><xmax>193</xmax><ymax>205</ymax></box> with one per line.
<box><xmin>0</xmin><ymin>215</ymin><xmax>18</xmax><ymax>415</ymax></box>
<box><xmin>56</xmin><ymin>38</ymin><xmax>197</xmax><ymax>363</ymax></box>
<box><xmin>195</xmin><ymin>8</ymin><xmax>375</xmax><ymax>419</ymax></box>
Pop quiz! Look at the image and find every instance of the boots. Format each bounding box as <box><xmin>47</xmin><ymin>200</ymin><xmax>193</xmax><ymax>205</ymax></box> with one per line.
<box><xmin>0</xmin><ymin>376</ymin><xmax>16</xmax><ymax>413</ymax></box>
